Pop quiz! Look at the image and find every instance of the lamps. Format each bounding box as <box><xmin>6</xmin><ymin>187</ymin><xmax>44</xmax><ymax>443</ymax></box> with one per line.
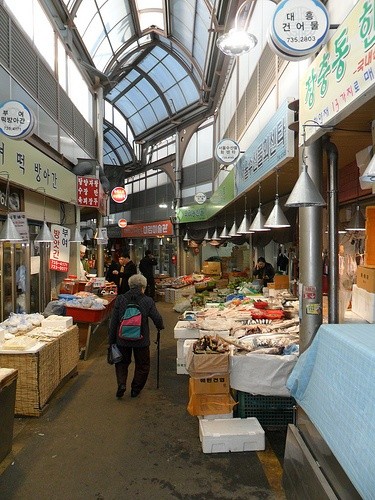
<box><xmin>284</xmin><ymin>120</ymin><xmax>330</xmax><ymax>208</ymax></box>
<box><xmin>33</xmin><ymin>187</ymin><xmax>103</xmax><ymax>244</ymax></box>
<box><xmin>183</xmin><ymin>231</ymin><xmax>191</xmax><ymax>241</ymax></box>
<box><xmin>0</xmin><ymin>171</ymin><xmax>24</xmax><ymax>242</ymax></box>
<box><xmin>216</xmin><ymin>0</ymin><xmax>279</xmax><ymax>57</ymax></box>
<box><xmin>202</xmin><ymin>169</ymin><xmax>291</xmax><ymax>241</ymax></box>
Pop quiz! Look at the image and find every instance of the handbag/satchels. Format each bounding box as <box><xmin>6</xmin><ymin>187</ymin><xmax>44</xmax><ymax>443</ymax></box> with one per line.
<box><xmin>108</xmin><ymin>343</ymin><xmax>122</xmax><ymax>365</ymax></box>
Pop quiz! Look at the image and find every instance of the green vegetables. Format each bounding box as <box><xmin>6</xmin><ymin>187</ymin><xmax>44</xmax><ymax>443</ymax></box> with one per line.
<box><xmin>226</xmin><ymin>277</ymin><xmax>254</xmax><ymax>288</ymax></box>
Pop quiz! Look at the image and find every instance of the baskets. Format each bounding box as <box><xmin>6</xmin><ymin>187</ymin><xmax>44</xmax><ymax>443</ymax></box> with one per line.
<box><xmin>238</xmin><ymin>390</ymin><xmax>296</xmax><ymax>409</ymax></box>
<box><xmin>238</xmin><ymin>410</ymin><xmax>295</xmax><ymax>430</ymax></box>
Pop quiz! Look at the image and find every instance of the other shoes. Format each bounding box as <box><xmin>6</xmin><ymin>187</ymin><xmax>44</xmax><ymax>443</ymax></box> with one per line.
<box><xmin>116</xmin><ymin>387</ymin><xmax>126</xmax><ymax>397</ymax></box>
<box><xmin>131</xmin><ymin>393</ymin><xmax>138</xmax><ymax>397</ymax></box>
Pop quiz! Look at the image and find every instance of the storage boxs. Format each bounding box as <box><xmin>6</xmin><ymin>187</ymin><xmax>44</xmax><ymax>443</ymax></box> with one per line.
<box><xmin>65</xmin><ymin>294</ymin><xmax>116</xmax><ymax>323</ymax></box>
<box><xmin>351</xmin><ymin>284</ymin><xmax>375</xmax><ymax>324</ymax></box>
<box><xmin>41</xmin><ymin>315</ymin><xmax>73</xmax><ymax>329</ymax></box>
<box><xmin>202</xmin><ymin>261</ymin><xmax>221</xmax><ymax>275</ymax></box>
<box><xmin>355</xmin><ymin>265</ymin><xmax>375</xmax><ymax>293</ymax></box>
<box><xmin>173</xmin><ymin>302</ymin><xmax>297</xmax><ymax>454</ymax></box>
<box><xmin>363</xmin><ymin>205</ymin><xmax>375</xmax><ymax>269</ymax></box>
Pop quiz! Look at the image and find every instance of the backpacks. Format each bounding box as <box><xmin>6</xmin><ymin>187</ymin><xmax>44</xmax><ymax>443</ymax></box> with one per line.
<box><xmin>118</xmin><ymin>293</ymin><xmax>146</xmax><ymax>341</ymax></box>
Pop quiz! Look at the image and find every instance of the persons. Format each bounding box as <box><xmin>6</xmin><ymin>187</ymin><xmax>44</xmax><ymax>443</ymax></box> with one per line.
<box><xmin>139</xmin><ymin>250</ymin><xmax>158</xmax><ymax>302</ymax></box>
<box><xmin>105</xmin><ymin>251</ymin><xmax>137</xmax><ymax>295</ymax></box>
<box><xmin>108</xmin><ymin>275</ymin><xmax>164</xmax><ymax>398</ymax></box>
<box><xmin>79</xmin><ymin>244</ymin><xmax>90</xmax><ymax>280</ymax></box>
<box><xmin>253</xmin><ymin>257</ymin><xmax>274</xmax><ymax>293</ymax></box>
